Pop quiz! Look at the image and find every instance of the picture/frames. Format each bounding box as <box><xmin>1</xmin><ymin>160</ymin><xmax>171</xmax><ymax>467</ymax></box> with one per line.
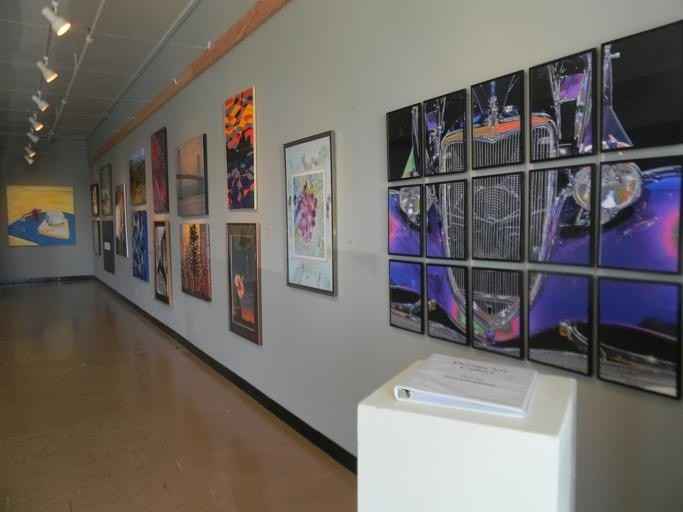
<box><xmin>281</xmin><ymin>128</ymin><xmax>336</xmax><ymax>299</ymax></box>
<box><xmin>89</xmin><ymin>183</ymin><xmax>99</xmax><ymax>217</ymax></box>
<box><xmin>90</xmin><ymin>219</ymin><xmax>101</xmax><ymax>256</ymax></box>
<box><xmin>152</xmin><ymin>218</ymin><xmax>172</xmax><ymax>308</ymax></box>
<box><xmin>224</xmin><ymin>220</ymin><xmax>263</xmax><ymax>348</ymax></box>
<box><xmin>99</xmin><ymin>162</ymin><xmax>112</xmax><ymax>217</ymax></box>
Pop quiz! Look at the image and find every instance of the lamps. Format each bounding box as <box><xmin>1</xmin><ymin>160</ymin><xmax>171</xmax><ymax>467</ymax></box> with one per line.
<box><xmin>23</xmin><ymin>5</ymin><xmax>72</xmax><ymax>165</ymax></box>
